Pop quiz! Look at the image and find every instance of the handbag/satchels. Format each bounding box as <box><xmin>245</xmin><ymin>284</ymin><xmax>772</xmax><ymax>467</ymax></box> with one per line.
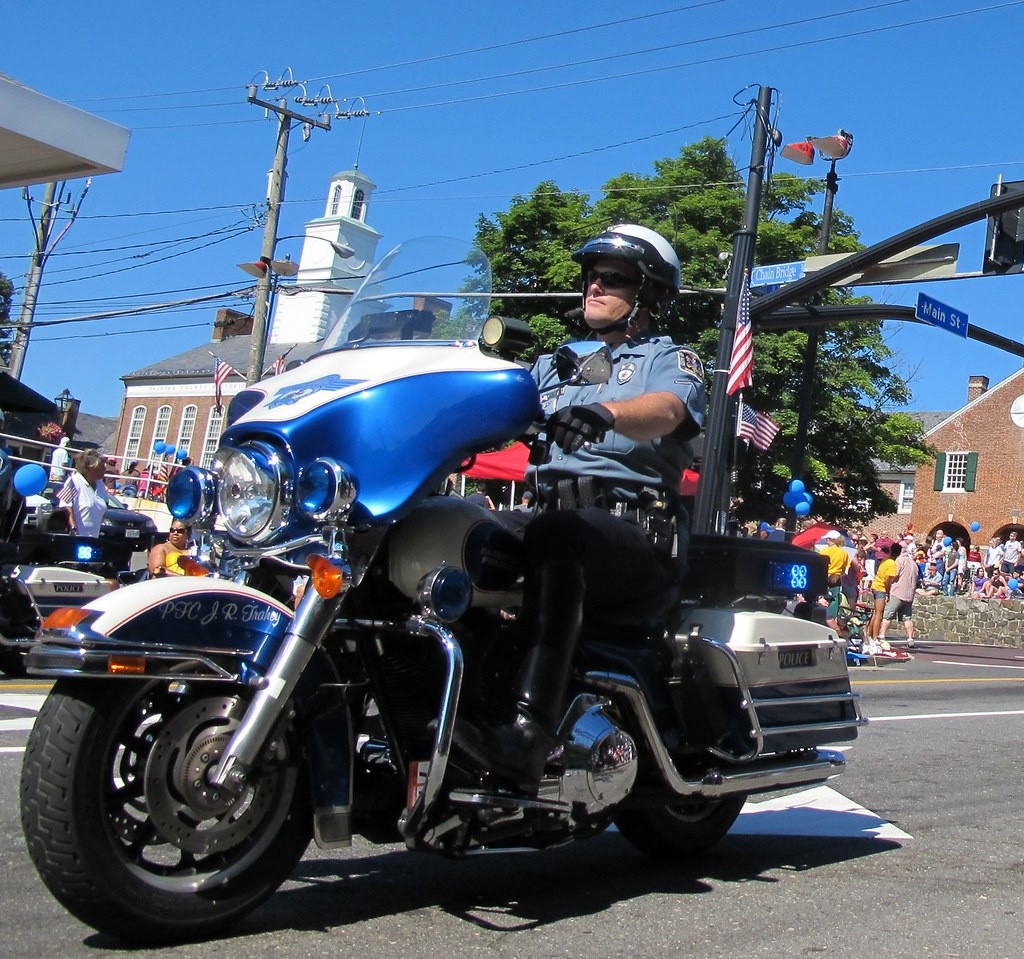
<box><xmin>123</xmin><ymin>485</ymin><xmax>136</xmax><ymax>498</ymax></box>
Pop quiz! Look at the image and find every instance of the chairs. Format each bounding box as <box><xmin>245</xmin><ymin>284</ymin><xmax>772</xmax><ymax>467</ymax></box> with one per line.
<box><xmin>829</xmin><ymin>587</ymin><xmax>871</xmax><ymax>649</ymax></box>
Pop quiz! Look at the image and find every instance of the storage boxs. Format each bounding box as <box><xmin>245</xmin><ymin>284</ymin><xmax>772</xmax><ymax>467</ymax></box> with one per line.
<box><xmin>11</xmin><ymin>563</ymin><xmax>106</xmax><ymax>615</ymax></box>
<box><xmin>677</xmin><ymin>608</ymin><xmax>857</xmax><ymax>751</ymax></box>
<box><xmin>100</xmin><ymin>508</ymin><xmax>158</xmax><ymax>553</ymax></box>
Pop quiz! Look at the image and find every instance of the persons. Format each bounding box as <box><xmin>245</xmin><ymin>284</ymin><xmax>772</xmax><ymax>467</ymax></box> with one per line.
<box><xmin>742</xmin><ymin>516</ymin><xmax>793</xmax><ymax>543</ymax></box>
<box><xmin>819</xmin><ymin>530</ymin><xmax>1024</xmax><ymax>646</ymax></box>
<box><xmin>0</xmin><ymin>410</ymin><xmax>208</xmax><ymax>579</ymax></box>
<box><xmin>445</xmin><ymin>474</ymin><xmax>536</xmax><ymax>513</ymax></box>
<box><xmin>426</xmin><ymin>223</ymin><xmax>708</xmax><ymax>800</ymax></box>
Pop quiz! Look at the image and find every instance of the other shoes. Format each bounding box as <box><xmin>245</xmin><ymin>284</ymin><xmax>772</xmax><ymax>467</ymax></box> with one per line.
<box><xmin>908</xmin><ymin>641</ymin><xmax>914</xmax><ymax>647</ymax></box>
<box><xmin>879</xmin><ymin>637</ymin><xmax>885</xmax><ymax>642</ymax></box>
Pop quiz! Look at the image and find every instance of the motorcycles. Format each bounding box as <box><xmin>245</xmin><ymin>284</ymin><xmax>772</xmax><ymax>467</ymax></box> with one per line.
<box><xmin>0</xmin><ymin>496</ymin><xmax>249</xmax><ymax>680</ymax></box>
<box><xmin>19</xmin><ymin>237</ymin><xmax>868</xmax><ymax>940</ymax></box>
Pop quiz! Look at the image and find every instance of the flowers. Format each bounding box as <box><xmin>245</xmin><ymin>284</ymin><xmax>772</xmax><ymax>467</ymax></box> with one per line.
<box><xmin>35</xmin><ymin>421</ymin><xmax>66</xmax><ymax>444</ymax></box>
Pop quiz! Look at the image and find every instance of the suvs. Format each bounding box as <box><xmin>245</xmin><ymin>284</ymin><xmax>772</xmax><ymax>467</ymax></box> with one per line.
<box><xmin>24</xmin><ymin>473</ymin><xmax>158</xmax><ymax>551</ymax></box>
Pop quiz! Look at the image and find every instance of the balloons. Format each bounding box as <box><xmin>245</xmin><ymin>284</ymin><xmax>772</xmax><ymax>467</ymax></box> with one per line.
<box><xmin>154</xmin><ymin>441</ymin><xmax>166</xmax><ymax>455</ymax></box>
<box><xmin>783</xmin><ymin>480</ymin><xmax>813</xmax><ymax>515</ymax></box>
<box><xmin>971</xmin><ymin>522</ymin><xmax>980</xmax><ymax>532</ymax></box>
<box><xmin>176</xmin><ymin>449</ymin><xmax>187</xmax><ymax>459</ymax></box>
<box><xmin>943</xmin><ymin>537</ymin><xmax>952</xmax><ymax>547</ymax></box>
<box><xmin>13</xmin><ymin>464</ymin><xmax>48</xmax><ymax>497</ymax></box>
<box><xmin>115</xmin><ymin>482</ymin><xmax>121</xmax><ymax>489</ymax></box>
<box><xmin>1007</xmin><ymin>579</ymin><xmax>1019</xmax><ymax>590</ymax></box>
<box><xmin>164</xmin><ymin>446</ymin><xmax>175</xmax><ymax>455</ymax></box>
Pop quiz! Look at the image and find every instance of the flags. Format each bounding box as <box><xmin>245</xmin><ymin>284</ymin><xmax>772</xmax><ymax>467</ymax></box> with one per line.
<box><xmin>736</xmin><ymin>401</ymin><xmax>782</xmax><ymax>451</ymax></box>
<box><xmin>725</xmin><ymin>273</ymin><xmax>752</xmax><ymax>397</ymax></box>
<box><xmin>272</xmin><ymin>347</ymin><xmax>292</xmax><ymax>376</ymax></box>
<box><xmin>55</xmin><ymin>478</ymin><xmax>78</xmax><ymax>504</ymax></box>
<box><xmin>214</xmin><ymin>356</ymin><xmax>234</xmax><ymax>417</ymax></box>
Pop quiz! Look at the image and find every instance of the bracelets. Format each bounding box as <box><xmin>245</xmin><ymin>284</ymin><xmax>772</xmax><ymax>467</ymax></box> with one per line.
<box><xmin>71</xmin><ymin>525</ymin><xmax>78</xmax><ymax>530</ymax></box>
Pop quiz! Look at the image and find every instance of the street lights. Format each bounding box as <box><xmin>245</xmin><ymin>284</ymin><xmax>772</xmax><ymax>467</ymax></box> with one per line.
<box><xmin>245</xmin><ymin>234</ymin><xmax>356</xmax><ymax>390</ymax></box>
<box><xmin>54</xmin><ymin>389</ymin><xmax>75</xmax><ymax>444</ymax></box>
<box><xmin>780</xmin><ymin>128</ymin><xmax>854</xmax><ymax>544</ymax></box>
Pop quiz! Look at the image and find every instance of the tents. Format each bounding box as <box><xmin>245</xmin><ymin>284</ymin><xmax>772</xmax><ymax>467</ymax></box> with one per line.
<box><xmin>457</xmin><ymin>440</ymin><xmax>699</xmax><ymax>521</ymax></box>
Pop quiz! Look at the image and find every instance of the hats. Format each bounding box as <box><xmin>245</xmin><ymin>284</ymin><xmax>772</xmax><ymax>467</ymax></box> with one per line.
<box><xmin>904</xmin><ymin>535</ymin><xmax>914</xmax><ymax>540</ymax></box>
<box><xmin>108</xmin><ymin>458</ymin><xmax>117</xmax><ymax>463</ymax></box>
<box><xmin>821</xmin><ymin>530</ymin><xmax>841</xmax><ymax>539</ymax></box>
<box><xmin>132</xmin><ymin>460</ymin><xmax>138</xmax><ymax>464</ymax></box>
<box><xmin>60</xmin><ymin>437</ymin><xmax>70</xmax><ymax>447</ymax></box>
<box><xmin>148</xmin><ymin>464</ymin><xmax>156</xmax><ymax>468</ymax></box>
<box><xmin>524</xmin><ymin>491</ymin><xmax>533</xmax><ymax>498</ymax></box>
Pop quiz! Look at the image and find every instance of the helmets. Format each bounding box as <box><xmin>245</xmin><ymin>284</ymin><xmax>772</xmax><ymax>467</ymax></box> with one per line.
<box><xmin>571</xmin><ymin>224</ymin><xmax>679</xmax><ymax>317</ymax></box>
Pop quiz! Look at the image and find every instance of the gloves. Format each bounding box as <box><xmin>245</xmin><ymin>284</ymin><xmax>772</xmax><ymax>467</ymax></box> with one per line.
<box><xmin>543</xmin><ymin>402</ymin><xmax>615</xmax><ymax>454</ymax></box>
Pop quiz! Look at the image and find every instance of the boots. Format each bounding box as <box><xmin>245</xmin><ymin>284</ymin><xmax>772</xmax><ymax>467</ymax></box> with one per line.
<box><xmin>425</xmin><ymin>598</ymin><xmax>582</xmax><ymax>799</ymax></box>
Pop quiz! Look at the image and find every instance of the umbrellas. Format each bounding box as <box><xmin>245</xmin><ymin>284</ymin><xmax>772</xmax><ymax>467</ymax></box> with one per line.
<box><xmin>792</xmin><ymin>522</ymin><xmax>842</xmax><ymax>549</ymax></box>
<box><xmin>815</xmin><ymin>530</ymin><xmax>858</xmax><ymax>576</ymax></box>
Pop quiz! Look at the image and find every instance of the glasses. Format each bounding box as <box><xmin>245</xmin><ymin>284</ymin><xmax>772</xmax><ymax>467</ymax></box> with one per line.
<box><xmin>170</xmin><ymin>528</ymin><xmax>188</xmax><ymax>533</ymax></box>
<box><xmin>586</xmin><ymin>268</ymin><xmax>638</xmax><ymax>288</ymax></box>
